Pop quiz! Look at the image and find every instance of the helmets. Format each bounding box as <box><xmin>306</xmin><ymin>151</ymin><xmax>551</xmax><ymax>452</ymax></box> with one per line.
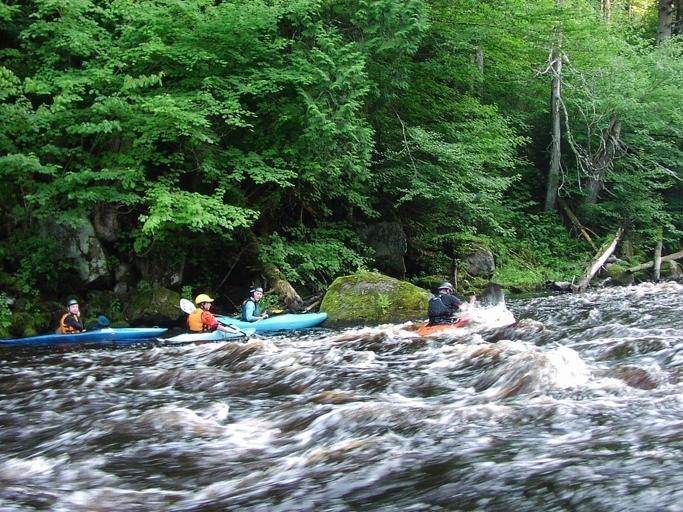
<box><xmin>248</xmin><ymin>285</ymin><xmax>263</xmax><ymax>302</ymax></box>
<box><xmin>437</xmin><ymin>283</ymin><xmax>454</xmax><ymax>295</ymax></box>
<box><xmin>67</xmin><ymin>299</ymin><xmax>79</xmax><ymax>306</ymax></box>
<box><xmin>195</xmin><ymin>294</ymin><xmax>214</xmax><ymax>305</ymax></box>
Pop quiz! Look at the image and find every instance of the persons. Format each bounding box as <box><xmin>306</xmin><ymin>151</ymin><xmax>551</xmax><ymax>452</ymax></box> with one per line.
<box><xmin>427</xmin><ymin>282</ymin><xmax>463</xmax><ymax>325</ymax></box>
<box><xmin>60</xmin><ymin>299</ymin><xmax>86</xmax><ymax>334</ymax></box>
<box><xmin>186</xmin><ymin>293</ymin><xmax>241</xmax><ymax>334</ymax></box>
<box><xmin>241</xmin><ymin>287</ymin><xmax>269</xmax><ymax>322</ymax></box>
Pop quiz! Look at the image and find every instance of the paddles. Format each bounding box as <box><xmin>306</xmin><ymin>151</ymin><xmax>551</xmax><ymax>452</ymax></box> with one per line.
<box><xmin>255</xmin><ymin>309</ymin><xmax>283</xmax><ymax>323</ymax></box>
<box><xmin>180</xmin><ymin>299</ymin><xmax>256</xmax><ymax>339</ymax></box>
<box><xmin>411</xmin><ymin>320</ymin><xmax>429</xmax><ymax>329</ymax></box>
<box><xmin>83</xmin><ymin>316</ymin><xmax>110</xmax><ymax>332</ymax></box>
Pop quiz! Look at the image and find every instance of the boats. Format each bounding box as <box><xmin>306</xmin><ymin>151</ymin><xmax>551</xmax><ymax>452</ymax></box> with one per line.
<box><xmin>334</xmin><ymin>319</ymin><xmax>471</xmax><ymax>342</ymax></box>
<box><xmin>1</xmin><ymin>327</ymin><xmax>169</xmax><ymax>349</ymax></box>
<box><xmin>164</xmin><ymin>312</ymin><xmax>328</xmax><ymax>347</ymax></box>
